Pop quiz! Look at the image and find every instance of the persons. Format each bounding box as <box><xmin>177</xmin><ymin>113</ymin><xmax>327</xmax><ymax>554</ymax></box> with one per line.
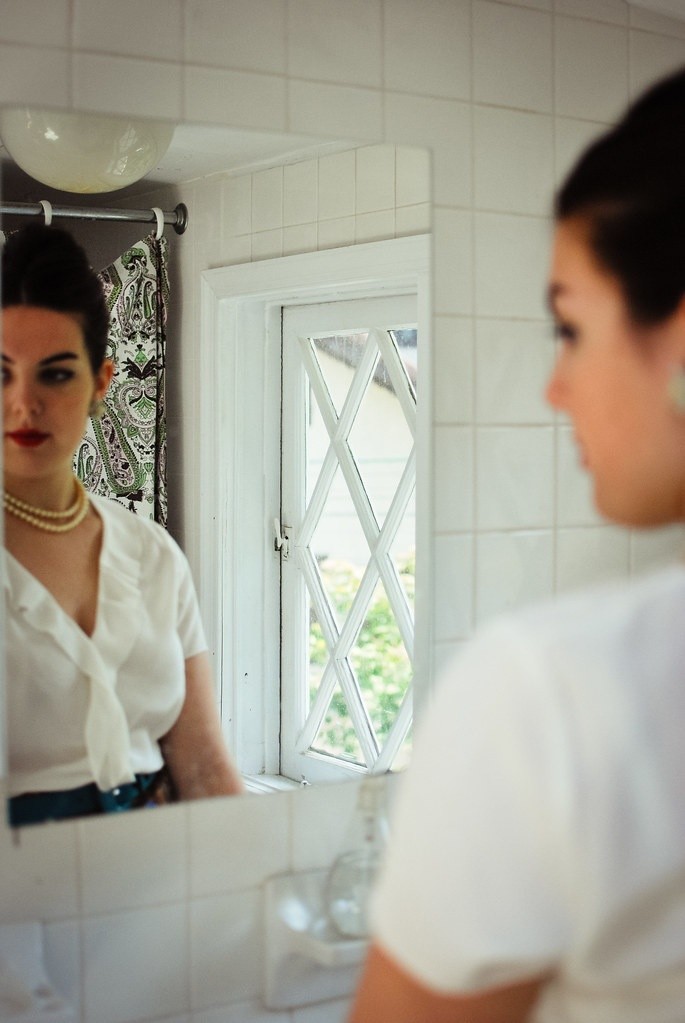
<box><xmin>0</xmin><ymin>224</ymin><xmax>245</xmax><ymax>831</ymax></box>
<box><xmin>352</xmin><ymin>61</ymin><xmax>685</xmax><ymax>1023</ymax></box>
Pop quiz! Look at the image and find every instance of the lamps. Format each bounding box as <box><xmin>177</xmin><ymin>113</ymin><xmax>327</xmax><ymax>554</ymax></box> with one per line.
<box><xmin>0</xmin><ymin>109</ymin><xmax>173</xmax><ymax>195</ymax></box>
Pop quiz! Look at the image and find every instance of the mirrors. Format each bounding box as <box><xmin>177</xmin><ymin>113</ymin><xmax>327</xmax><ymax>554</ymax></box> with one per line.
<box><xmin>0</xmin><ymin>104</ymin><xmax>434</xmax><ymax>833</ymax></box>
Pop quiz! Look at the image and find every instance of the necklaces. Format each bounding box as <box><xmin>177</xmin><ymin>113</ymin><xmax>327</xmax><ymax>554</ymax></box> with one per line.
<box><xmin>3</xmin><ymin>476</ymin><xmax>91</xmax><ymax>532</ymax></box>
